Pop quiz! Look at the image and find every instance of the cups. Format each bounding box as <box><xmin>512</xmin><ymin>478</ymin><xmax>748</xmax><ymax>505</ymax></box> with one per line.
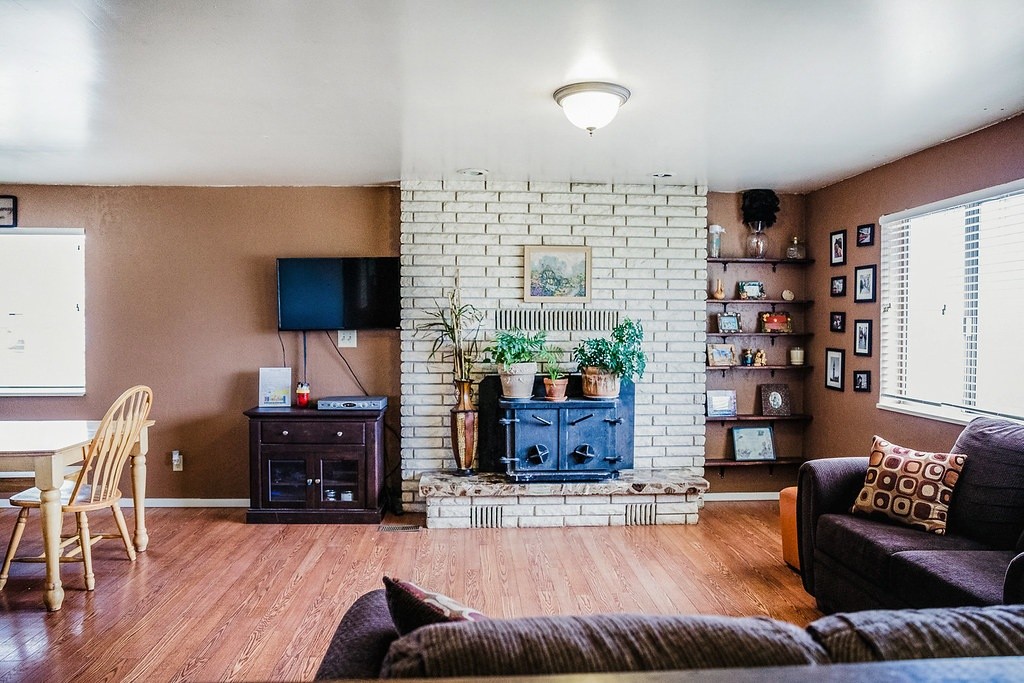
<box><xmin>791</xmin><ymin>348</ymin><xmax>804</xmax><ymax>365</ymax></box>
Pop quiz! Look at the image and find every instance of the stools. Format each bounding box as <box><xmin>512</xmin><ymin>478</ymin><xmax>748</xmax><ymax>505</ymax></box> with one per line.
<box><xmin>779</xmin><ymin>486</ymin><xmax>799</xmax><ymax>574</ymax></box>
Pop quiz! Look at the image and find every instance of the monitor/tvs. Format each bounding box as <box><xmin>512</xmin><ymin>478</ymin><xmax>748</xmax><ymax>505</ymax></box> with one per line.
<box><xmin>276</xmin><ymin>257</ymin><xmax>403</xmax><ymax>331</ymax></box>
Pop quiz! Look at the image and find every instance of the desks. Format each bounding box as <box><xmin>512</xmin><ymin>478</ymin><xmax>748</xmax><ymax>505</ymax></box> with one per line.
<box><xmin>0</xmin><ymin>419</ymin><xmax>149</xmax><ymax>612</ymax></box>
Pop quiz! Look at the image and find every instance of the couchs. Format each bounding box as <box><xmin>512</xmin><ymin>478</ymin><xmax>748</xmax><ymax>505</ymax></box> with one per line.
<box><xmin>303</xmin><ymin>415</ymin><xmax>1024</xmax><ymax>683</ymax></box>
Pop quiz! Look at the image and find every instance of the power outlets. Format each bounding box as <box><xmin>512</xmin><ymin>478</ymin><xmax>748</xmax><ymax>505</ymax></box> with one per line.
<box><xmin>337</xmin><ymin>330</ymin><xmax>357</xmax><ymax>348</ymax></box>
<box><xmin>173</xmin><ymin>457</ymin><xmax>184</xmax><ymax>471</ymax></box>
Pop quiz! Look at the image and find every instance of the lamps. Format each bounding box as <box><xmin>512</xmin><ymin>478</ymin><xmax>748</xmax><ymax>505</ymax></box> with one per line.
<box><xmin>552</xmin><ymin>81</ymin><xmax>631</xmax><ymax>134</ymax></box>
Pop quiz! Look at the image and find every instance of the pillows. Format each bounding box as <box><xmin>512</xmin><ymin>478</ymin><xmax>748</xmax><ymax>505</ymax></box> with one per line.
<box><xmin>381</xmin><ymin>573</ymin><xmax>489</xmax><ymax>638</ymax></box>
<box><xmin>853</xmin><ymin>433</ymin><xmax>969</xmax><ymax>538</ymax></box>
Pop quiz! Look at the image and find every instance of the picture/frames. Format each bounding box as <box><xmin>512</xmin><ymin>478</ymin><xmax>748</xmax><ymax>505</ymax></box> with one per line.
<box><xmin>522</xmin><ymin>244</ymin><xmax>591</xmax><ymax>304</ymax></box>
<box><xmin>825</xmin><ymin>348</ymin><xmax>845</xmax><ymax>393</ymax></box>
<box><xmin>717</xmin><ymin>312</ymin><xmax>743</xmax><ymax>335</ymax></box>
<box><xmin>853</xmin><ymin>370</ymin><xmax>871</xmax><ymax>393</ymax></box>
<box><xmin>829</xmin><ymin>311</ymin><xmax>846</xmax><ymax>332</ymax></box>
<box><xmin>707</xmin><ymin>389</ymin><xmax>738</xmax><ymax>416</ymax></box>
<box><xmin>829</xmin><ymin>230</ymin><xmax>847</xmax><ymax>266</ymax></box>
<box><xmin>831</xmin><ymin>275</ymin><xmax>847</xmax><ymax>296</ymax></box>
<box><xmin>853</xmin><ymin>319</ymin><xmax>872</xmax><ymax>356</ymax></box>
<box><xmin>707</xmin><ymin>342</ymin><xmax>737</xmax><ymax>367</ymax></box>
<box><xmin>856</xmin><ymin>224</ymin><xmax>874</xmax><ymax>247</ymax></box>
<box><xmin>730</xmin><ymin>425</ymin><xmax>777</xmax><ymax>463</ymax></box>
<box><xmin>854</xmin><ymin>265</ymin><xmax>877</xmax><ymax>304</ymax></box>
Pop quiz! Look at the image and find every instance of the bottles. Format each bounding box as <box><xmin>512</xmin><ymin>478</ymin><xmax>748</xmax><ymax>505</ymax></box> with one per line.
<box><xmin>744</xmin><ymin>349</ymin><xmax>753</xmax><ymax>366</ymax></box>
<box><xmin>710</xmin><ymin>225</ymin><xmax>721</xmax><ymax>258</ymax></box>
<box><xmin>747</xmin><ymin>231</ymin><xmax>769</xmax><ymax>259</ymax></box>
<box><xmin>787</xmin><ymin>235</ymin><xmax>804</xmax><ymax>259</ymax></box>
<box><xmin>714</xmin><ymin>279</ymin><xmax>725</xmax><ymax>299</ymax></box>
<box><xmin>324</xmin><ymin>489</ymin><xmax>353</xmax><ymax>502</ymax></box>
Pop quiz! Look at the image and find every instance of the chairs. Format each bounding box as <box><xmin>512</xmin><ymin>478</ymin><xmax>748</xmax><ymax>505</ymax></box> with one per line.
<box><xmin>0</xmin><ymin>384</ymin><xmax>154</xmax><ymax>592</ymax></box>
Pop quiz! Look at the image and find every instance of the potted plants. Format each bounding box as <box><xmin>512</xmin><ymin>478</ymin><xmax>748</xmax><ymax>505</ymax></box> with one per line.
<box><xmin>742</xmin><ymin>190</ymin><xmax>781</xmax><ymax>258</ymax></box>
<box><xmin>487</xmin><ymin>329</ymin><xmax>543</xmax><ymax>400</ymax></box>
<box><xmin>431</xmin><ymin>298</ymin><xmax>483</xmax><ymax>474</ymax></box>
<box><xmin>543</xmin><ymin>348</ymin><xmax>568</xmax><ymax>402</ymax></box>
<box><xmin>572</xmin><ymin>318</ymin><xmax>648</xmax><ymax>399</ymax></box>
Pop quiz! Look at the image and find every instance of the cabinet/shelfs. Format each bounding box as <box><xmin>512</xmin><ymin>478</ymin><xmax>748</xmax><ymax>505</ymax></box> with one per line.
<box><xmin>706</xmin><ymin>255</ymin><xmax>814</xmax><ymax>476</ymax></box>
<box><xmin>242</xmin><ymin>400</ymin><xmax>387</xmax><ymax>525</ymax></box>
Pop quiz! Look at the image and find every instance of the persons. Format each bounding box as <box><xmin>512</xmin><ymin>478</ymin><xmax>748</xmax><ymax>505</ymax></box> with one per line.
<box><xmin>835</xmin><ymin>243</ymin><xmax>842</xmax><ymax>257</ymax></box>
<box><xmin>857</xmin><ymin>376</ymin><xmax>862</xmax><ymax>388</ymax></box>
